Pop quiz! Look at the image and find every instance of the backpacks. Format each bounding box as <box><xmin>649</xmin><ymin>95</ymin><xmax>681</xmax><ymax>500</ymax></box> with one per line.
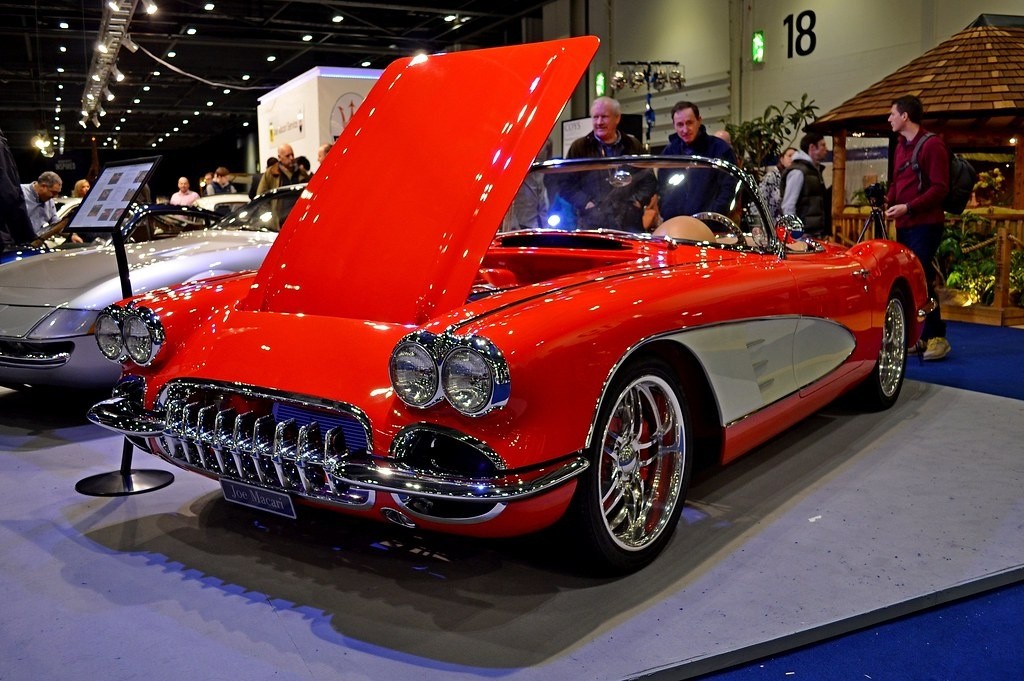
<box><xmin>911</xmin><ymin>132</ymin><xmax>978</xmax><ymax>216</ymax></box>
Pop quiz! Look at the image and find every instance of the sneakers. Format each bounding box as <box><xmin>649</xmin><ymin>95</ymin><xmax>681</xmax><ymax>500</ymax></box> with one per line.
<box><xmin>905</xmin><ymin>339</ymin><xmax>927</xmax><ymax>356</ymax></box>
<box><xmin>922</xmin><ymin>337</ymin><xmax>953</xmax><ymax>362</ymax></box>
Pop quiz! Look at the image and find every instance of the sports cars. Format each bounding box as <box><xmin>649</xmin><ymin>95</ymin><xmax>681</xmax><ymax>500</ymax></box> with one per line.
<box><xmin>86</xmin><ymin>36</ymin><xmax>928</xmax><ymax>569</ymax></box>
<box><xmin>0</xmin><ymin>182</ymin><xmax>308</xmax><ymax>392</ymax></box>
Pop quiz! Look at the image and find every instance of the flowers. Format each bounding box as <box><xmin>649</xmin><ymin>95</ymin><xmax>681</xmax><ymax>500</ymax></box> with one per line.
<box><xmin>972</xmin><ymin>168</ymin><xmax>1008</xmax><ymax>206</ymax></box>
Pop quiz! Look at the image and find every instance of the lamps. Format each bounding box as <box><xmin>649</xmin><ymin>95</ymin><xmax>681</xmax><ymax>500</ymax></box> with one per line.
<box><xmin>92</xmin><ymin>68</ymin><xmax>104</xmax><ymax>82</ymax></box>
<box><xmin>109</xmin><ymin>0</ymin><xmax>125</xmax><ymax>11</ymax></box>
<box><xmin>102</xmin><ymin>88</ymin><xmax>115</xmax><ymax>102</ymax></box>
<box><xmin>98</xmin><ymin>35</ymin><xmax>114</xmax><ymax>53</ymax></box>
<box><xmin>142</xmin><ymin>0</ymin><xmax>158</xmax><ymax>14</ymax></box>
<box><xmin>111</xmin><ymin>67</ymin><xmax>125</xmax><ymax>82</ymax></box>
<box><xmin>81</xmin><ymin>104</ymin><xmax>92</xmax><ymax>116</ymax></box>
<box><xmin>87</xmin><ymin>87</ymin><xmax>98</xmax><ymax>100</ymax></box>
<box><xmin>120</xmin><ymin>26</ymin><xmax>139</xmax><ymax>53</ymax></box>
<box><xmin>92</xmin><ymin>113</ymin><xmax>100</xmax><ymax>127</ymax></box>
<box><xmin>79</xmin><ymin>116</ymin><xmax>89</xmax><ymax>125</ymax></box>
<box><xmin>35</xmin><ymin>130</ymin><xmax>55</xmax><ymax>158</ymax></box>
<box><xmin>96</xmin><ymin>104</ymin><xmax>106</xmax><ymax>117</ymax></box>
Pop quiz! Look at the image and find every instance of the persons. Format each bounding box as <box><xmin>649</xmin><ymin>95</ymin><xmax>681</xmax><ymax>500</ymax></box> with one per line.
<box><xmin>249</xmin><ymin>144</ymin><xmax>336</xmax><ymax>228</ymax></box>
<box><xmin>659</xmin><ymin>101</ymin><xmax>744</xmax><ymax>234</ymax></box>
<box><xmin>884</xmin><ymin>94</ymin><xmax>951</xmax><ymax>361</ymax></box>
<box><xmin>554</xmin><ymin>96</ymin><xmax>658</xmax><ymax>234</ymax></box>
<box><xmin>500</xmin><ymin>136</ymin><xmax>554</xmax><ymax>232</ymax></box>
<box><xmin>20</xmin><ymin>171</ymin><xmax>90</xmax><ymax>246</ymax></box>
<box><xmin>758</xmin><ymin>132</ymin><xmax>834</xmax><ymax>239</ymax></box>
<box><xmin>168</xmin><ymin>168</ymin><xmax>239</xmax><ymax>228</ymax></box>
